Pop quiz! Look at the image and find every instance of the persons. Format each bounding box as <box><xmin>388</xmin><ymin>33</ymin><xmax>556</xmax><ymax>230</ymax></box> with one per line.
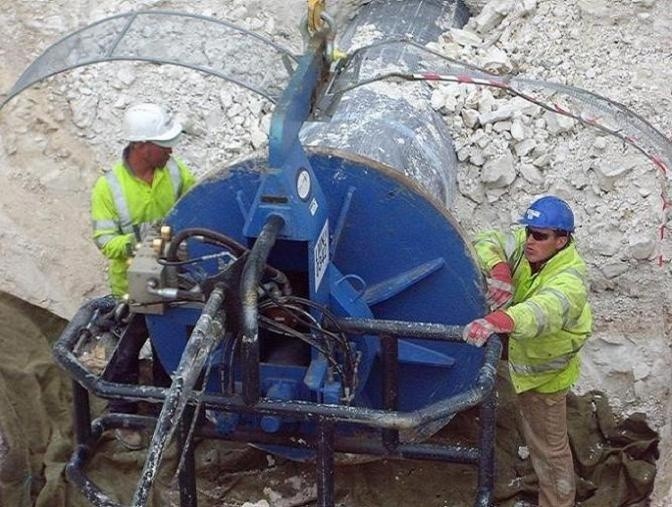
<box><xmin>89</xmin><ymin>102</ymin><xmax>195</xmax><ymax>453</ymax></box>
<box><xmin>462</xmin><ymin>193</ymin><xmax>594</xmax><ymax>506</ymax></box>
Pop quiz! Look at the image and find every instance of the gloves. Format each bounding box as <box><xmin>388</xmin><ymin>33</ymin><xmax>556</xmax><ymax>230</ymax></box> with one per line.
<box><xmin>485</xmin><ymin>263</ymin><xmax>515</xmax><ymax>311</ymax></box>
<box><xmin>463</xmin><ymin>311</ymin><xmax>513</xmax><ymax>347</ymax></box>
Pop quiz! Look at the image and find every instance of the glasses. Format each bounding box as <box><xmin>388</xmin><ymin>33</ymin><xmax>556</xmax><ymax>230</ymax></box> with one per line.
<box><xmin>526</xmin><ymin>227</ymin><xmax>556</xmax><ymax>240</ymax></box>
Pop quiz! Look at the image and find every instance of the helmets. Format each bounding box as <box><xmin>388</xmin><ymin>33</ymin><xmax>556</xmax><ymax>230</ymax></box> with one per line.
<box><xmin>518</xmin><ymin>195</ymin><xmax>574</xmax><ymax>233</ymax></box>
<box><xmin>120</xmin><ymin>102</ymin><xmax>183</xmax><ymax>143</ymax></box>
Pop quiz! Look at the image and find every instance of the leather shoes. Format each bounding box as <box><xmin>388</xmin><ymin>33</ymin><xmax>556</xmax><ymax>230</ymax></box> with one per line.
<box><xmin>113</xmin><ymin>427</ymin><xmax>143</xmax><ymax>449</ymax></box>
